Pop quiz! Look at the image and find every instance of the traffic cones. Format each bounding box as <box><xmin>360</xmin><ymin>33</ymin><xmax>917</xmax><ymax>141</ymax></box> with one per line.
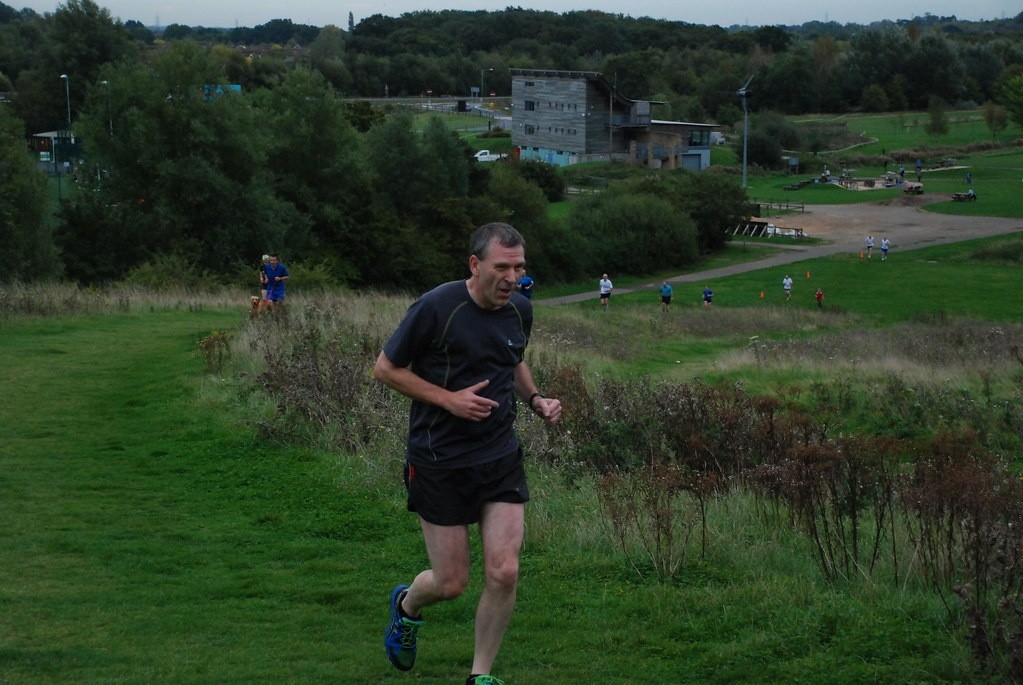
<box><xmin>806</xmin><ymin>271</ymin><xmax>810</xmax><ymax>279</ymax></box>
<box><xmin>760</xmin><ymin>291</ymin><xmax>764</xmax><ymax>298</ymax></box>
<box><xmin>859</xmin><ymin>250</ymin><xmax>863</xmax><ymax>258</ymax></box>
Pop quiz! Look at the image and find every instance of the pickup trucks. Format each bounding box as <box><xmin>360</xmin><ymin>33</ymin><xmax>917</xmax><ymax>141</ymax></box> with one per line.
<box><xmin>474</xmin><ymin>150</ymin><xmax>500</xmax><ymax>162</ymax></box>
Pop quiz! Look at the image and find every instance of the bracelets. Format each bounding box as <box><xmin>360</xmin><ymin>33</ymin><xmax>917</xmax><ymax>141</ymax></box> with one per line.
<box><xmin>528</xmin><ymin>392</ymin><xmax>544</xmax><ymax>409</ymax></box>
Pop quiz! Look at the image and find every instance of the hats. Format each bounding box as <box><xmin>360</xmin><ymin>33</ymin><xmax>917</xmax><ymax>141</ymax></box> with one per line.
<box><xmin>261</xmin><ymin>255</ymin><xmax>269</xmax><ymax>262</ymax></box>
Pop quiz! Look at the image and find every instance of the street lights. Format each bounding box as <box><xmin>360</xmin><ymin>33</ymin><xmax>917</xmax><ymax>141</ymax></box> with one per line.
<box><xmin>480</xmin><ymin>68</ymin><xmax>494</xmax><ymax>96</ymax></box>
<box><xmin>101</xmin><ymin>80</ymin><xmax>113</xmax><ymax>142</ymax></box>
<box><xmin>59</xmin><ymin>74</ymin><xmax>71</xmax><ymax>123</ymax></box>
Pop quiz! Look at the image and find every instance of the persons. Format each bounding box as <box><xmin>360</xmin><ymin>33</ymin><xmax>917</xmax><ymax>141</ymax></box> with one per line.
<box><xmin>260</xmin><ymin>255</ymin><xmax>289</xmax><ymax>304</ymax></box>
<box><xmin>600</xmin><ymin>274</ymin><xmax>613</xmax><ymax>312</ymax></box>
<box><xmin>968</xmin><ymin>187</ymin><xmax>973</xmax><ymax>197</ymax></box>
<box><xmin>701</xmin><ymin>284</ymin><xmax>714</xmax><ymax>306</ymax></box>
<box><xmin>783</xmin><ymin>274</ymin><xmax>793</xmax><ymax>300</ymax></box>
<box><xmin>881</xmin><ymin>237</ymin><xmax>890</xmax><ymax>260</ymax></box>
<box><xmin>899</xmin><ymin>166</ymin><xmax>905</xmax><ymax>173</ymax></box>
<box><xmin>865</xmin><ymin>235</ymin><xmax>874</xmax><ymax>258</ymax></box>
<box><xmin>816</xmin><ymin>288</ymin><xmax>823</xmax><ymax>311</ymax></box>
<box><xmin>660</xmin><ymin>282</ymin><xmax>673</xmax><ymax>313</ymax></box>
<box><xmin>916</xmin><ymin>159</ymin><xmax>922</xmax><ymax>181</ymax></box>
<box><xmin>374</xmin><ymin>222</ymin><xmax>562</xmax><ymax>685</ymax></box>
<box><xmin>516</xmin><ymin>270</ymin><xmax>534</xmax><ymax>299</ymax></box>
<box><xmin>824</xmin><ymin>167</ymin><xmax>830</xmax><ymax>177</ymax></box>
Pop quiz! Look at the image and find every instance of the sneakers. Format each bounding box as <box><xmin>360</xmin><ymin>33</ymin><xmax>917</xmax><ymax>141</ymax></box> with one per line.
<box><xmin>465</xmin><ymin>673</ymin><xmax>503</xmax><ymax>685</ymax></box>
<box><xmin>384</xmin><ymin>586</ymin><xmax>425</xmax><ymax>671</ymax></box>
